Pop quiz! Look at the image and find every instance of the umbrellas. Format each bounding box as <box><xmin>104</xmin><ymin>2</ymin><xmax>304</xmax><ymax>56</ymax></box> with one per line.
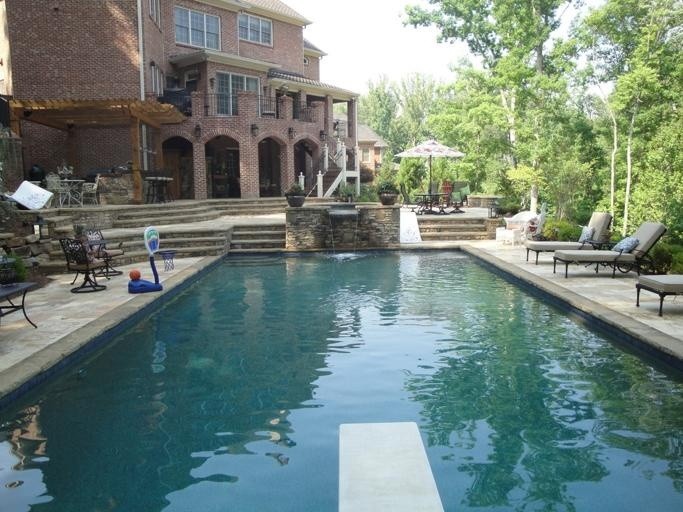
<box><xmin>393</xmin><ymin>140</ymin><xmax>466</xmax><ymax>195</ymax></box>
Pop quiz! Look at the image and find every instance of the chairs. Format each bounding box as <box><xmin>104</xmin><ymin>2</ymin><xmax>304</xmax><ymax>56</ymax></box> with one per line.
<box><xmin>400</xmin><ymin>182</ymin><xmax>467</xmax><ymax>215</ymax></box>
<box><xmin>46</xmin><ymin>165</ymin><xmax>99</xmax><ymax>208</ymax></box>
<box><xmin>504</xmin><ymin>211</ymin><xmax>683</xmax><ymax>316</ymax></box>
<box><xmin>0</xmin><ymin>225</ymin><xmax>123</xmax><ymax>329</ymax></box>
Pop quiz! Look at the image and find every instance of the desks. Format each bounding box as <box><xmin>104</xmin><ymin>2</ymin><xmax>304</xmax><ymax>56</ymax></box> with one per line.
<box><xmin>496</xmin><ymin>227</ymin><xmax>522</xmax><ymax>250</ymax></box>
<box><xmin>144</xmin><ymin>177</ymin><xmax>175</xmax><ymax>204</ymax></box>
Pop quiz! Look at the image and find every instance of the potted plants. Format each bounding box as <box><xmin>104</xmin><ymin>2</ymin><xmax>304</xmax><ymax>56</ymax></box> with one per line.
<box><xmin>377</xmin><ymin>182</ymin><xmax>400</xmax><ymax>205</ymax></box>
<box><xmin>285</xmin><ymin>184</ymin><xmax>306</xmax><ymax>207</ymax></box>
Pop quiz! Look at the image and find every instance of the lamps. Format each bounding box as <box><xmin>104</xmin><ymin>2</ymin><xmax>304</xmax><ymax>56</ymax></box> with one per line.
<box><xmin>210</xmin><ymin>77</ymin><xmax>217</xmax><ymax>90</ymax></box>
<box><xmin>320</xmin><ymin>130</ymin><xmax>328</xmax><ymax>141</ymax></box>
<box><xmin>251</xmin><ymin>124</ymin><xmax>258</xmax><ymax>137</ymax></box>
<box><xmin>288</xmin><ymin>127</ymin><xmax>296</xmax><ymax>140</ymax></box>
<box><xmin>194</xmin><ymin>125</ymin><xmax>201</xmax><ymax>143</ymax></box>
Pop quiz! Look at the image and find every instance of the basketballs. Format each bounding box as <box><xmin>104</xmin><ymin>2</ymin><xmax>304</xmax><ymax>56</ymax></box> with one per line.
<box><xmin>130</xmin><ymin>269</ymin><xmax>141</xmax><ymax>280</ymax></box>
